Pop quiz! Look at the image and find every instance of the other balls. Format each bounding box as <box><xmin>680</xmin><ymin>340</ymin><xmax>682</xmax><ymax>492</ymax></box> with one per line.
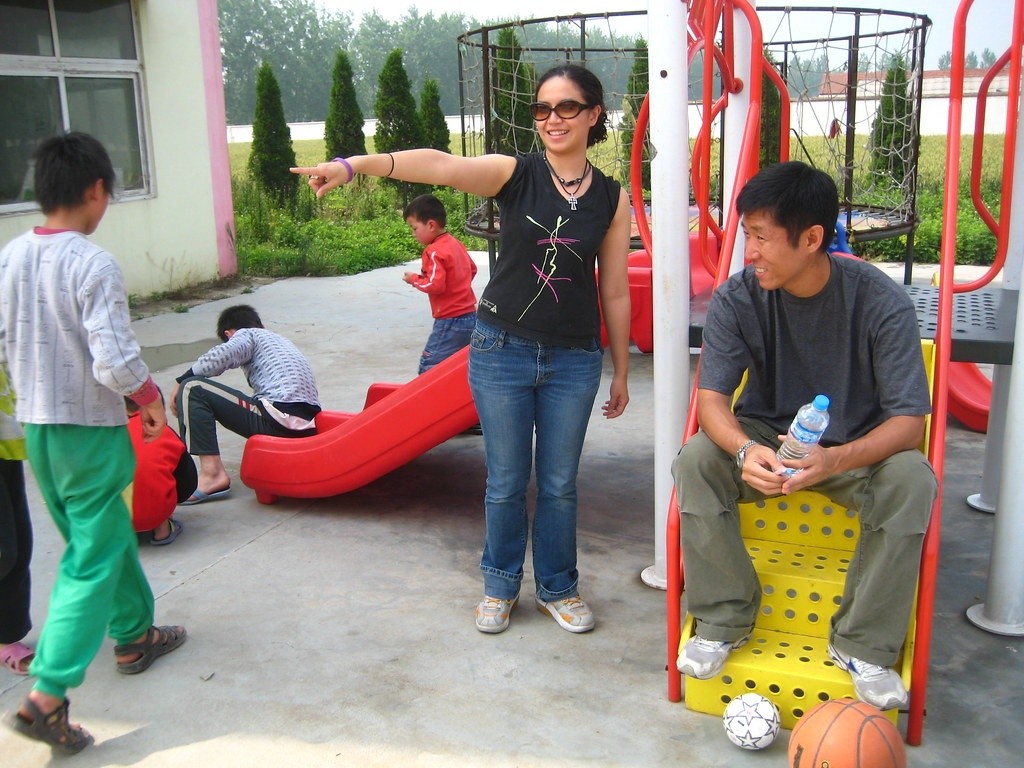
<box><xmin>723</xmin><ymin>693</ymin><xmax>781</xmax><ymax>750</ymax></box>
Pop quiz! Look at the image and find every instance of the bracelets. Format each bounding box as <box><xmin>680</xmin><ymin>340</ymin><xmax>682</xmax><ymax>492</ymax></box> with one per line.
<box><xmin>386</xmin><ymin>153</ymin><xmax>394</xmax><ymax>178</ymax></box>
<box><xmin>331</xmin><ymin>157</ymin><xmax>353</xmax><ymax>184</ymax></box>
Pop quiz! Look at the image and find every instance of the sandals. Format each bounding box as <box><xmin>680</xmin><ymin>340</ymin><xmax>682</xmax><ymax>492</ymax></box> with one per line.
<box><xmin>2</xmin><ymin>693</ymin><xmax>90</xmax><ymax>754</ymax></box>
<box><xmin>115</xmin><ymin>623</ymin><xmax>187</xmax><ymax>675</ymax></box>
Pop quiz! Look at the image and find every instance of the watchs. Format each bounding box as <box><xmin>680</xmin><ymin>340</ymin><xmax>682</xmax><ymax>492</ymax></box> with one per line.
<box><xmin>736</xmin><ymin>441</ymin><xmax>758</xmax><ymax>468</ymax></box>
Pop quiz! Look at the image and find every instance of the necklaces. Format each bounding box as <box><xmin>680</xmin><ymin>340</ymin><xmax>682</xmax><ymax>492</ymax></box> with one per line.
<box><xmin>542</xmin><ymin>148</ymin><xmax>593</xmax><ymax>209</ymax></box>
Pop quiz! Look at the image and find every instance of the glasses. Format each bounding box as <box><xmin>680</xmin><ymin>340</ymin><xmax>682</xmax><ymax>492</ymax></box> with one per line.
<box><xmin>531</xmin><ymin>100</ymin><xmax>594</xmax><ymax>121</ymax></box>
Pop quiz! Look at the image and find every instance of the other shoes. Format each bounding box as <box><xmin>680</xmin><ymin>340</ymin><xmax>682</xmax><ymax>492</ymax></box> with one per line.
<box><xmin>463</xmin><ymin>422</ymin><xmax>483</xmax><ymax>436</ymax></box>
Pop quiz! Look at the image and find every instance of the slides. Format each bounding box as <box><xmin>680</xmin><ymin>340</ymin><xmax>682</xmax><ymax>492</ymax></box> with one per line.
<box><xmin>947</xmin><ymin>362</ymin><xmax>993</xmax><ymax>434</ymax></box>
<box><xmin>238</xmin><ymin>248</ymin><xmax>653</xmax><ymax>504</ymax></box>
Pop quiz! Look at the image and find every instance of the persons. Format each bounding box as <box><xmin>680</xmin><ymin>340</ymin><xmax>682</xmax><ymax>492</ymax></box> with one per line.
<box><xmin>0</xmin><ymin>132</ymin><xmax>187</xmax><ymax>757</ymax></box>
<box><xmin>402</xmin><ymin>192</ymin><xmax>478</xmax><ymax>374</ymax></box>
<box><xmin>170</xmin><ymin>305</ymin><xmax>322</xmax><ymax>506</ymax></box>
<box><xmin>124</xmin><ymin>383</ymin><xmax>199</xmax><ymax>546</ymax></box>
<box><xmin>290</xmin><ymin>59</ymin><xmax>631</xmax><ymax>634</ymax></box>
<box><xmin>670</xmin><ymin>161</ymin><xmax>938</xmax><ymax>709</ymax></box>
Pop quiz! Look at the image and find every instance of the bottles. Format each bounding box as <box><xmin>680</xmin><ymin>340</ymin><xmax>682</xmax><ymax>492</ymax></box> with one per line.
<box><xmin>776</xmin><ymin>395</ymin><xmax>830</xmax><ymax>480</ymax></box>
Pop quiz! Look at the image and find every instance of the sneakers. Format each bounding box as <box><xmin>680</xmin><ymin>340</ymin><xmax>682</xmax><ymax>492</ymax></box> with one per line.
<box><xmin>828</xmin><ymin>641</ymin><xmax>908</xmax><ymax>710</ymax></box>
<box><xmin>535</xmin><ymin>590</ymin><xmax>595</xmax><ymax>632</ymax></box>
<box><xmin>476</xmin><ymin>586</ymin><xmax>519</xmax><ymax>633</ymax></box>
<box><xmin>676</xmin><ymin>630</ymin><xmax>754</xmax><ymax>679</ymax></box>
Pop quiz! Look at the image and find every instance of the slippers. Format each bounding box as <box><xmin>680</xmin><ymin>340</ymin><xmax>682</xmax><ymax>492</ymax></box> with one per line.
<box><xmin>0</xmin><ymin>641</ymin><xmax>37</xmax><ymax>675</ymax></box>
<box><xmin>180</xmin><ymin>485</ymin><xmax>232</xmax><ymax>505</ymax></box>
<box><xmin>150</xmin><ymin>519</ymin><xmax>182</xmax><ymax>544</ymax></box>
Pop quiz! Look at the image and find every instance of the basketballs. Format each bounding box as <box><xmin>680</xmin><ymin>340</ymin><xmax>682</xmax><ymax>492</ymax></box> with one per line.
<box><xmin>787</xmin><ymin>699</ymin><xmax>908</xmax><ymax>768</ymax></box>
